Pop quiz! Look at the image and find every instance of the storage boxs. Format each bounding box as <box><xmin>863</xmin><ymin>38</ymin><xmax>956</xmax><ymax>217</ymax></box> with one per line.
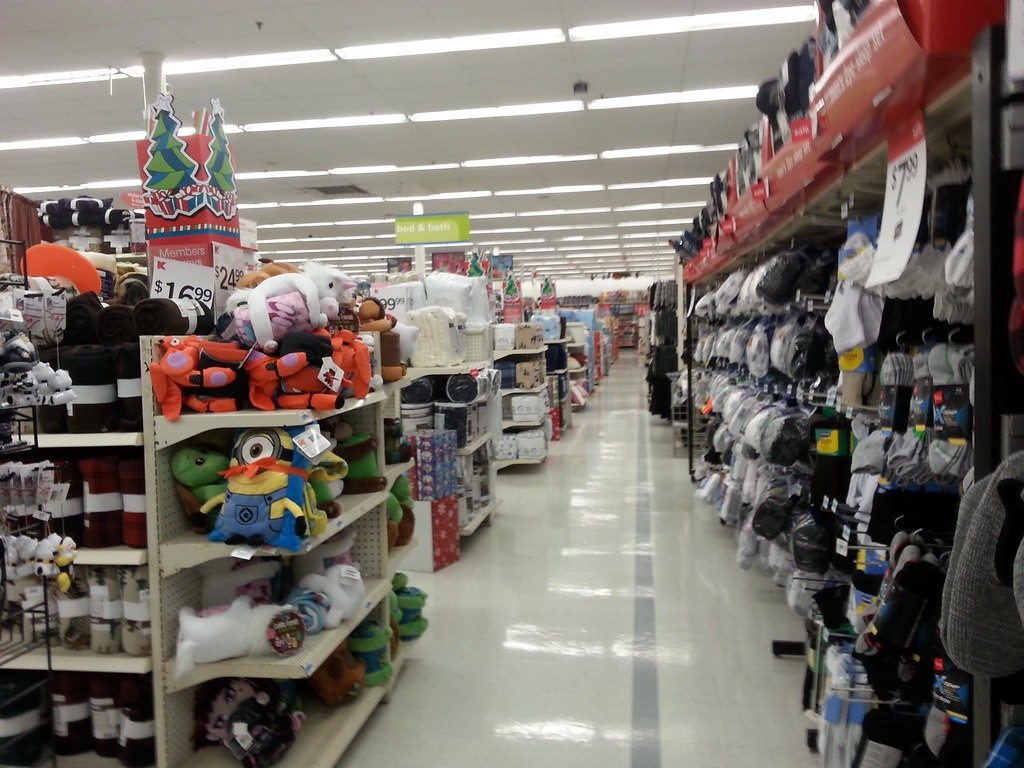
<box><xmin>496</xmin><ymin>322</ymin><xmax>544</xmax><ymax>351</ymax></box>
<box><xmin>389</xmin><ymin>429</ymin><xmax>459</xmax><ymax>574</ymax></box>
<box><xmin>495</xmin><ymin>358</ymin><xmax>590</xmax><ymax>461</ymax></box>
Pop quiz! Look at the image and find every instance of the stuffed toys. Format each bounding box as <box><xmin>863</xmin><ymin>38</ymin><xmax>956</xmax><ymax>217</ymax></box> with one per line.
<box><xmin>389</xmin><ymin>572</ymin><xmax>429</xmax><ymax>641</ymax></box>
<box><xmin>173</xmin><ymin>526</ymin><xmax>366</xmax><ymax>679</ymax></box>
<box><xmin>190</xmin><ymin>623</ymin><xmax>393</xmax><ymax>768</ymax></box>
<box><xmin>383</xmin><ymin>418</ymin><xmax>413</xmax><ymax>464</ymax></box>
<box><xmin>149</xmin><ymin>259</ymin><xmax>407</xmax><ymax>423</ymax></box>
<box><xmin>170</xmin><ymin>416</ymin><xmax>386</xmax><ymax>552</ymax></box>
<box><xmin>387</xmin><ymin>474</ymin><xmax>416</xmax><ymax>551</ymax></box>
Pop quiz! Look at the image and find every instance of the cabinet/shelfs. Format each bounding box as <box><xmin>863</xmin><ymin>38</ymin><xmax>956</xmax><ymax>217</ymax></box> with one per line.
<box><xmin>385</xmin><ymin>324</ymin><xmax>505</xmax><ymax>537</ymax></box>
<box><xmin>0</xmin><ymin>238</ymin><xmax>416</xmax><ymax>768</ymax></box>
<box><xmin>544</xmin><ymin>298</ymin><xmax>650</xmax><ymax>438</ymax></box>
<box><xmin>492</xmin><ymin>343</ymin><xmax>551</xmax><ymax>473</ymax></box>
